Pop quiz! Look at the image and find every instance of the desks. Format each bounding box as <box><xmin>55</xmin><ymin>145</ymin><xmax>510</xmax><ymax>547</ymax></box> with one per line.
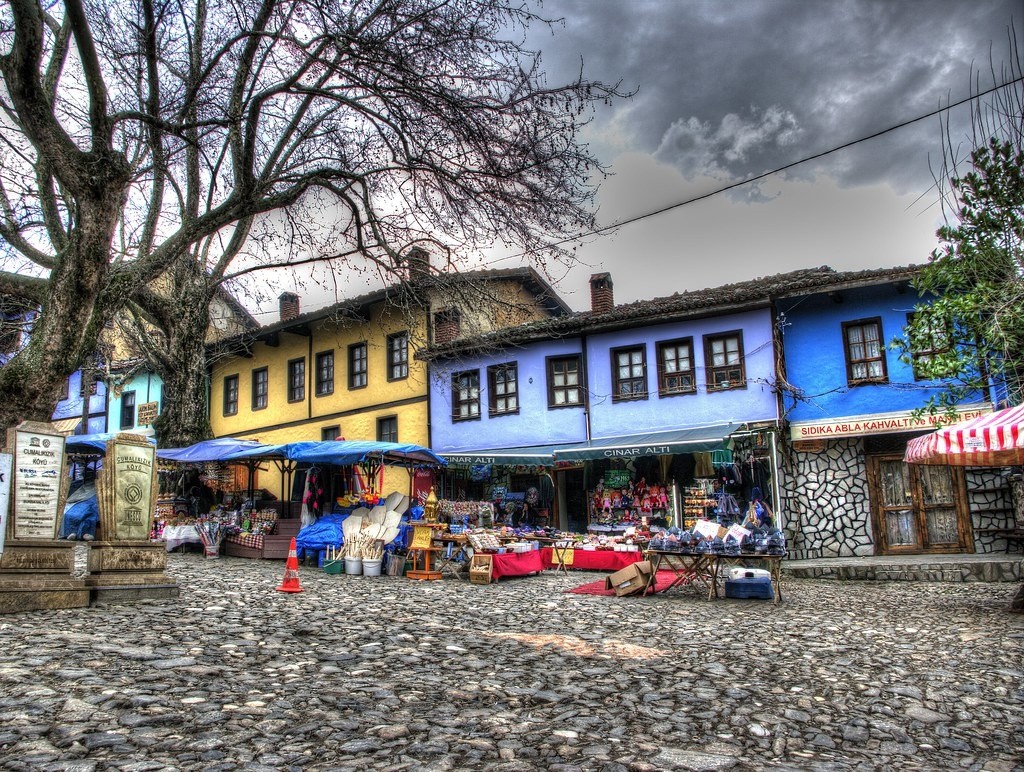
<box><xmin>541</xmin><ymin>547</ymin><xmax>644</xmax><ymax>571</ymax></box>
<box><xmin>432</xmin><ymin>538</ymin><xmax>471</xmax><ymax>581</ymax></box>
<box><xmin>475</xmin><ymin>549</ymin><xmax>546</xmax><ymax>584</ymax></box>
<box><xmin>517</xmin><ymin>537</ymin><xmax>578</xmax><ymax>577</ymax></box>
<box><xmin>641</xmin><ymin>550</ymin><xmax>784</xmax><ymax>607</ymax></box>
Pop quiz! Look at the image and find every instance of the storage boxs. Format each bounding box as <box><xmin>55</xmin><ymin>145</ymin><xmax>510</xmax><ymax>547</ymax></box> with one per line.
<box><xmin>605</xmin><ymin>560</ymin><xmax>658</xmax><ymax>597</ymax></box>
<box><xmin>693</xmin><ymin>519</ymin><xmax>728</xmax><ymax>539</ymax></box>
<box><xmin>722</xmin><ymin>523</ymin><xmax>751</xmax><ymax>544</ymax></box>
<box><xmin>469</xmin><ymin>554</ymin><xmax>493</xmax><ymax>585</ymax></box>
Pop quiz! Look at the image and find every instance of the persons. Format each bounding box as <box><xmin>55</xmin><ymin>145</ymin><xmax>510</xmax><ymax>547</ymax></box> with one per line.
<box><xmin>592</xmin><ymin>476</ymin><xmax>669</xmax><ymax>526</ymax></box>
<box><xmin>518</xmin><ymin>502</ymin><xmax>537</xmax><ymax>526</ymax></box>
<box><xmin>494</xmin><ymin>504</ymin><xmax>506</xmax><ymax>523</ymax></box>
<box><xmin>503</xmin><ymin>502</ymin><xmax>520</xmax><ymax>528</ymax></box>
<box><xmin>713</xmin><ymin>480</ymin><xmax>724</xmax><ymax>500</ymax></box>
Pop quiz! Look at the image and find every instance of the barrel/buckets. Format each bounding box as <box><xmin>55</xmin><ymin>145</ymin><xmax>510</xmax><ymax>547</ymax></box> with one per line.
<box><xmin>387</xmin><ymin>553</ymin><xmax>406</xmax><ymax>576</ymax></box>
<box><xmin>362</xmin><ymin>559</ymin><xmax>382</xmax><ymax>576</ymax></box>
<box><xmin>344</xmin><ymin>557</ymin><xmax>362</xmax><ymax>574</ymax></box>
<box><xmin>321</xmin><ymin>560</ymin><xmax>342</xmax><ymax>574</ymax></box>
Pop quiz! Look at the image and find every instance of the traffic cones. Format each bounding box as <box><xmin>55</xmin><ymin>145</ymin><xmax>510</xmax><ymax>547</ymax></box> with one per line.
<box><xmin>276</xmin><ymin>537</ymin><xmax>305</xmax><ymax>593</ymax></box>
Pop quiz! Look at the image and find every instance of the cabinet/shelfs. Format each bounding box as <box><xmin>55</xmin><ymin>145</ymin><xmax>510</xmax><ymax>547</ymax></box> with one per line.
<box><xmin>586</xmin><ymin>489</ymin><xmax>666</xmax><ymax>535</ymax></box>
<box><xmin>679</xmin><ymin>486</ymin><xmax>708</xmax><ymax>531</ymax></box>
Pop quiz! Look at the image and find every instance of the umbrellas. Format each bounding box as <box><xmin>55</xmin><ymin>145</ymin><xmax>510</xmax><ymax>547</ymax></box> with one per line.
<box><xmin>903</xmin><ymin>402</ymin><xmax>1024</xmax><ymax>465</ymax></box>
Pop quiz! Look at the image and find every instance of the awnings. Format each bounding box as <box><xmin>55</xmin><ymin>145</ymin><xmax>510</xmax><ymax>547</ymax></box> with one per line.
<box><xmin>555</xmin><ymin>422</ymin><xmax>745</xmax><ymax>461</ymax></box>
<box><xmin>66</xmin><ymin>434</ymin><xmax>156</xmax><ymax>482</ymax></box>
<box><xmin>156</xmin><ymin>438</ymin><xmax>313</xmax><ymax>520</ymax></box>
<box><xmin>434</xmin><ymin>443</ymin><xmax>582</xmax><ymax>465</ymax></box>
<box><xmin>289</xmin><ymin>440</ymin><xmax>447</xmax><ymax>522</ymax></box>
